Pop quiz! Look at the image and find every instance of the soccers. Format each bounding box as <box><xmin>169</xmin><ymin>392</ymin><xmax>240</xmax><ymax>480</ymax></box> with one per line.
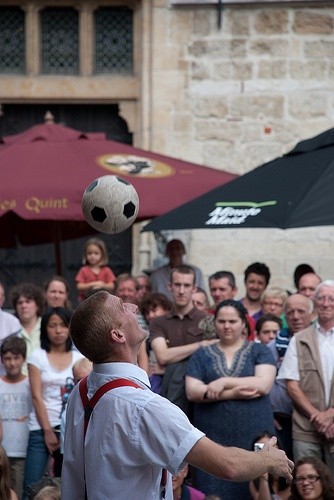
<box><xmin>82</xmin><ymin>175</ymin><xmax>140</xmax><ymax>233</ymax></box>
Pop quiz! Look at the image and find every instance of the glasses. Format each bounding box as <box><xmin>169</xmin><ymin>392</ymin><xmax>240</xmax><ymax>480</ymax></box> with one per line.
<box><xmin>293</xmin><ymin>474</ymin><xmax>321</xmax><ymax>484</ymax></box>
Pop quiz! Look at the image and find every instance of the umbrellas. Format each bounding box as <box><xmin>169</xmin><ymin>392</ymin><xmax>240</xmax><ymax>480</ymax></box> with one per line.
<box><xmin>141</xmin><ymin>128</ymin><xmax>334</xmax><ymax>233</ymax></box>
<box><xmin>0</xmin><ymin>112</ymin><xmax>249</xmax><ymax>280</ymax></box>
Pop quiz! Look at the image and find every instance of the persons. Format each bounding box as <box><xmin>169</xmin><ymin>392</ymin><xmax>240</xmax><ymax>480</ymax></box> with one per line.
<box><xmin>147</xmin><ymin>237</ymin><xmax>207</xmax><ymax>304</ymax></box>
<box><xmin>75</xmin><ymin>234</ymin><xmax>116</xmax><ymax>304</ymax></box>
<box><xmin>0</xmin><ymin>264</ymin><xmax>334</xmax><ymax>500</ymax></box>
